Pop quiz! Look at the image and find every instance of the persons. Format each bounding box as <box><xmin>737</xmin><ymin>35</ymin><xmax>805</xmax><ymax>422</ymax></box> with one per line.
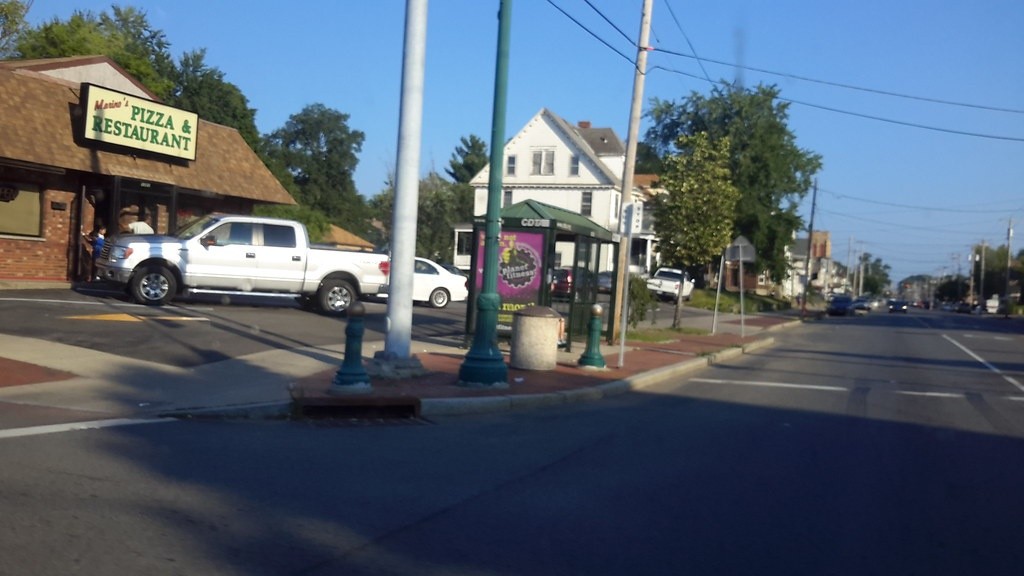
<box><xmin>80</xmin><ymin>225</ymin><xmax>106</xmax><ymax>282</ymax></box>
<box><xmin>121</xmin><ymin>214</ymin><xmax>155</xmax><ymax>235</ymax></box>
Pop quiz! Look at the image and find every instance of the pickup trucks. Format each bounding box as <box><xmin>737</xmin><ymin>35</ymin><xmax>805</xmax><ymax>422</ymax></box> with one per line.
<box><xmin>94</xmin><ymin>212</ymin><xmax>392</xmax><ymax>319</ymax></box>
<box><xmin>646</xmin><ymin>267</ymin><xmax>696</xmax><ymax>304</ymax></box>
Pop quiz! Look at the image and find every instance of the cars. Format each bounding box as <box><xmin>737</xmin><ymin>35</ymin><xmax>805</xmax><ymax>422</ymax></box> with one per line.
<box><xmin>854</xmin><ymin>296</ymin><xmax>887</xmax><ymax>310</ymax></box>
<box><xmin>889</xmin><ymin>300</ymin><xmax>908</xmax><ymax>314</ymax></box>
<box><xmin>430</xmin><ymin>264</ymin><xmax>469</xmax><ymax>281</ymax></box>
<box><xmin>551</xmin><ymin>266</ymin><xmax>573</xmax><ymax>298</ymax></box>
<box><xmin>359</xmin><ymin>255</ymin><xmax>470</xmax><ymax>309</ymax></box>
<box><xmin>938</xmin><ymin>301</ymin><xmax>971</xmax><ymax>315</ymax></box>
<box><xmin>828</xmin><ymin>294</ymin><xmax>856</xmax><ymax>317</ymax></box>
<box><xmin>597</xmin><ymin>270</ymin><xmax>613</xmax><ymax>294</ymax></box>
